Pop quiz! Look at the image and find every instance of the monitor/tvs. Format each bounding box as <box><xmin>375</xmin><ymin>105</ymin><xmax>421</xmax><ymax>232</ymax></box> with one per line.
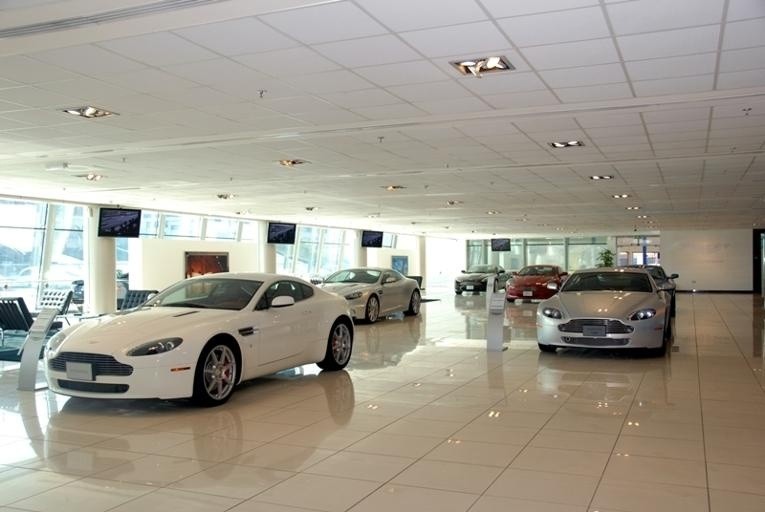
<box><xmin>268</xmin><ymin>223</ymin><xmax>295</xmax><ymax>243</ymax></box>
<box><xmin>492</xmin><ymin>239</ymin><xmax>510</xmax><ymax>251</ymax></box>
<box><xmin>98</xmin><ymin>208</ymin><xmax>141</xmax><ymax>236</ymax></box>
<box><xmin>362</xmin><ymin>231</ymin><xmax>383</xmax><ymax>247</ymax></box>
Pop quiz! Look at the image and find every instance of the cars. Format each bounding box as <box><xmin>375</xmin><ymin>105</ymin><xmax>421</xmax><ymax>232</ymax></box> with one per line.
<box><xmin>43</xmin><ymin>272</ymin><xmax>355</xmax><ymax>408</ymax></box>
<box><xmin>505</xmin><ymin>264</ymin><xmax>570</xmax><ymax>302</ymax></box>
<box><xmin>317</xmin><ymin>267</ymin><xmax>421</xmax><ymax>324</ymax></box>
<box><xmin>536</xmin><ymin>265</ymin><xmax>679</xmax><ymax>357</ymax></box>
<box><xmin>455</xmin><ymin>264</ymin><xmax>513</xmax><ymax>295</ymax></box>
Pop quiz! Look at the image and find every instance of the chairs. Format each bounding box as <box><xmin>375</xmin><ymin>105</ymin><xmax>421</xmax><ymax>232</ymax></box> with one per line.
<box><xmin>584</xmin><ymin>275</ymin><xmax>647</xmax><ymax>289</ymax></box>
<box><xmin>0</xmin><ymin>288</ymin><xmax>164</xmax><ymax>358</ymax></box>
<box><xmin>209</xmin><ymin>283</ymin><xmax>292</xmax><ymax>309</ymax></box>
